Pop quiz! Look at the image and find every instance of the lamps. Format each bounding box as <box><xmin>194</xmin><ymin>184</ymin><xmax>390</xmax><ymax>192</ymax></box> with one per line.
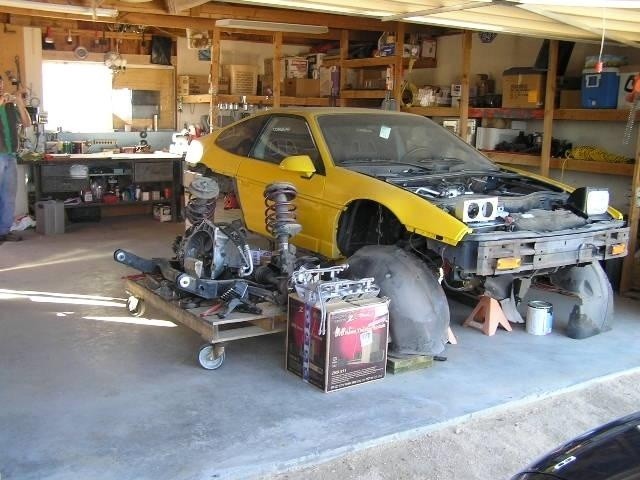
<box><xmin>104</xmin><ymin>24</ymin><xmax>128</xmax><ymax>74</ymax></box>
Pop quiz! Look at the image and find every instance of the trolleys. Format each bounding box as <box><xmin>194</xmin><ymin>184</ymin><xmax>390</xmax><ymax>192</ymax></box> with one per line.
<box><xmin>121</xmin><ymin>273</ymin><xmax>379</xmax><ymax>372</ymax></box>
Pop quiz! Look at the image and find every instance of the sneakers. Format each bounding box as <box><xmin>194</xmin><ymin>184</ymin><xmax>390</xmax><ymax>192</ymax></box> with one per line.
<box><xmin>1</xmin><ymin>233</ymin><xmax>21</xmax><ymax>241</ymax></box>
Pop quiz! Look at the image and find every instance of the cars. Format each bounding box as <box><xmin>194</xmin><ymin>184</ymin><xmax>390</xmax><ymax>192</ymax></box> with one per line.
<box><xmin>182</xmin><ymin>107</ymin><xmax>631</xmax><ymax>314</ymax></box>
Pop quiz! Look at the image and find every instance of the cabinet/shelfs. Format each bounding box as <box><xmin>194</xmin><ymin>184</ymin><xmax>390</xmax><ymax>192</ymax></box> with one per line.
<box><xmin>176</xmin><ymin>0</ymin><xmax>640</xmax><ymax>303</ymax></box>
<box><xmin>22</xmin><ymin>151</ymin><xmax>184</xmax><ymax>222</ymax></box>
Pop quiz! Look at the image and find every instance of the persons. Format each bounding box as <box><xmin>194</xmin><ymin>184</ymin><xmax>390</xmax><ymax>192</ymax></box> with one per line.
<box><xmin>0</xmin><ymin>76</ymin><xmax>32</xmax><ymax>246</ymax></box>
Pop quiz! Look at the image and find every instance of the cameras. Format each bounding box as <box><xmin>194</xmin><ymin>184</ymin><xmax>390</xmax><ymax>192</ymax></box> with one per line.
<box><xmin>7</xmin><ymin>95</ymin><xmax>16</xmax><ymax>102</ymax></box>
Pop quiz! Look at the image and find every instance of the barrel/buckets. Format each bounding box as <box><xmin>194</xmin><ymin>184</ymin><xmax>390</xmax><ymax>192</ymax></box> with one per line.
<box><xmin>526</xmin><ymin>300</ymin><xmax>553</xmax><ymax>336</ymax></box>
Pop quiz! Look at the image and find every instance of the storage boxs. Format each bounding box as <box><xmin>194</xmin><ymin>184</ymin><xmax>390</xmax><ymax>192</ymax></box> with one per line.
<box><xmin>288</xmin><ymin>291</ymin><xmax>389</xmax><ymax>395</ymax></box>
